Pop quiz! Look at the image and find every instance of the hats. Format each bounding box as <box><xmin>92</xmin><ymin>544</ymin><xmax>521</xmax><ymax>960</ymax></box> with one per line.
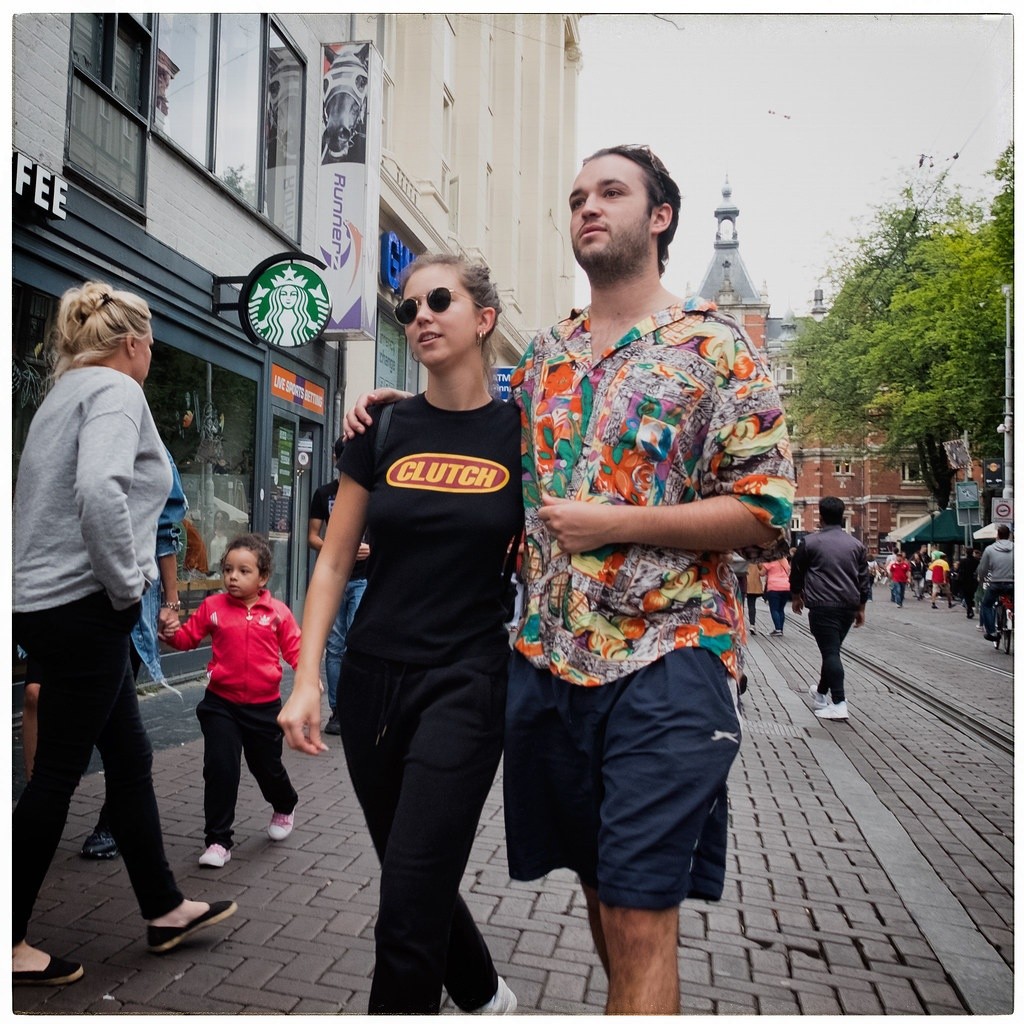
<box><xmin>897</xmin><ymin>553</ymin><xmax>902</xmax><ymax>556</ymax></box>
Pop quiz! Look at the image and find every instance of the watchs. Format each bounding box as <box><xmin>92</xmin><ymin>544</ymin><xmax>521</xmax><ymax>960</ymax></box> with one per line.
<box><xmin>162</xmin><ymin>601</ymin><xmax>181</xmax><ymax>611</ymax></box>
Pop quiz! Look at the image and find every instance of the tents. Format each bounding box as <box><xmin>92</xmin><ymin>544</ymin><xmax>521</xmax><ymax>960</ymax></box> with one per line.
<box><xmin>885</xmin><ymin>503</ymin><xmax>979</xmax><ymax>544</ymax></box>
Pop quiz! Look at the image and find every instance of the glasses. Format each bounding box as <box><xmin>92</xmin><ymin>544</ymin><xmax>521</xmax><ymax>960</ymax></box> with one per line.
<box><xmin>394</xmin><ymin>287</ymin><xmax>486</xmax><ymax>325</ymax></box>
<box><xmin>584</xmin><ymin>144</ymin><xmax>667</xmax><ymax>195</ymax></box>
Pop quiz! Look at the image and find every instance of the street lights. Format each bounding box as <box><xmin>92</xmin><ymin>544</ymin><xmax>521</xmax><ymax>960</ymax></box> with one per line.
<box><xmin>1001</xmin><ymin>283</ymin><xmax>1014</xmax><ymax>542</ymax></box>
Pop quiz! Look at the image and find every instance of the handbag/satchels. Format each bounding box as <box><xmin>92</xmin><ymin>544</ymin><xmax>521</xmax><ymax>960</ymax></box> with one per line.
<box><xmin>925</xmin><ymin>569</ymin><xmax>933</xmax><ymax>581</ymax></box>
<box><xmin>913</xmin><ymin>572</ymin><xmax>922</xmax><ymax>579</ymax></box>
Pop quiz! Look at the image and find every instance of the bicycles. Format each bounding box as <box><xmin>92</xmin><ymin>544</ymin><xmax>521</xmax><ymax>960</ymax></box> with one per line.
<box><xmin>982</xmin><ymin>580</ymin><xmax>1014</xmax><ymax>655</ymax></box>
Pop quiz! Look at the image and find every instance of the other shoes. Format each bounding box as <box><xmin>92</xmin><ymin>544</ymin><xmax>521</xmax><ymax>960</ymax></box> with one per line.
<box><xmin>976</xmin><ymin>625</ymin><xmax>984</xmax><ymax>630</ymax></box>
<box><xmin>897</xmin><ymin>604</ymin><xmax>901</xmax><ymax>608</ymax></box>
<box><xmin>918</xmin><ymin>595</ymin><xmax>922</xmax><ymax>600</ymax></box>
<box><xmin>966</xmin><ymin>613</ymin><xmax>974</xmax><ymax>619</ymax></box>
<box><xmin>12</xmin><ymin>954</ymin><xmax>83</xmax><ymax>984</ymax></box>
<box><xmin>146</xmin><ymin>901</ymin><xmax>238</xmax><ymax>952</ymax></box>
<box><xmin>932</xmin><ymin>605</ymin><xmax>937</xmax><ymax>608</ymax></box>
<box><xmin>325</xmin><ymin>713</ymin><xmax>340</xmax><ymax>735</ymax></box>
<box><xmin>867</xmin><ymin>599</ymin><xmax>872</xmax><ymax>603</ymax></box>
<box><xmin>983</xmin><ymin>634</ymin><xmax>998</xmax><ymax>641</ymax></box>
<box><xmin>948</xmin><ymin>603</ymin><xmax>956</xmax><ymax>608</ymax></box>
<box><xmin>770</xmin><ymin>630</ymin><xmax>783</xmax><ymax>636</ymax></box>
<box><xmin>749</xmin><ymin>627</ymin><xmax>756</xmax><ymax>635</ymax></box>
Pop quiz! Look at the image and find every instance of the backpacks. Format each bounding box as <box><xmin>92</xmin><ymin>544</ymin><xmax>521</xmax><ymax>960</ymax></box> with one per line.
<box><xmin>868</xmin><ymin>562</ymin><xmax>876</xmax><ymax>576</ymax></box>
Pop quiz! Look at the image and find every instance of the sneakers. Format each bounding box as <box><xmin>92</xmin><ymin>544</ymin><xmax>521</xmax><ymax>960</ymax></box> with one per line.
<box><xmin>462</xmin><ymin>976</ymin><xmax>518</xmax><ymax>1015</ymax></box>
<box><xmin>808</xmin><ymin>685</ymin><xmax>826</xmax><ymax>705</ymax></box>
<box><xmin>81</xmin><ymin>830</ymin><xmax>120</xmax><ymax>860</ymax></box>
<box><xmin>199</xmin><ymin>843</ymin><xmax>231</xmax><ymax>867</ymax></box>
<box><xmin>268</xmin><ymin>807</ymin><xmax>295</xmax><ymax>840</ymax></box>
<box><xmin>814</xmin><ymin>701</ymin><xmax>849</xmax><ymax>719</ymax></box>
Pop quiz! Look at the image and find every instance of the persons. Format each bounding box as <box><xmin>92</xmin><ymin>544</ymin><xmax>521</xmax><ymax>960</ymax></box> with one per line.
<box><xmin>789</xmin><ymin>548</ymin><xmax>798</xmax><ymax>558</ymax></box>
<box><xmin>276</xmin><ymin>250</ymin><xmax>526</xmax><ymax>1015</ymax></box>
<box><xmin>788</xmin><ymin>497</ymin><xmax>871</xmax><ymax>720</ymax></box>
<box><xmin>182</xmin><ymin>510</ymin><xmax>249</xmax><ymax>580</ymax></box>
<box><xmin>309</xmin><ymin>432</ymin><xmax>374</xmax><ymax>736</ymax></box>
<box><xmin>977</xmin><ymin>525</ymin><xmax>1014</xmax><ymax>642</ymax></box>
<box><xmin>760</xmin><ymin>553</ymin><xmax>792</xmax><ymax>637</ymax></box>
<box><xmin>729</xmin><ymin>551</ymin><xmax>764</xmax><ymax>635</ymax></box>
<box><xmin>864</xmin><ymin>543</ymin><xmax>995</xmax><ymax>619</ymax></box>
<box><xmin>12</xmin><ymin>281</ymin><xmax>238</xmax><ymax>989</ymax></box>
<box><xmin>12</xmin><ymin>326</ymin><xmax>189</xmax><ymax>860</ymax></box>
<box><xmin>157</xmin><ymin>533</ymin><xmax>323</xmax><ymax>869</ymax></box>
<box><xmin>342</xmin><ymin>146</ymin><xmax>794</xmax><ymax>1015</ymax></box>
